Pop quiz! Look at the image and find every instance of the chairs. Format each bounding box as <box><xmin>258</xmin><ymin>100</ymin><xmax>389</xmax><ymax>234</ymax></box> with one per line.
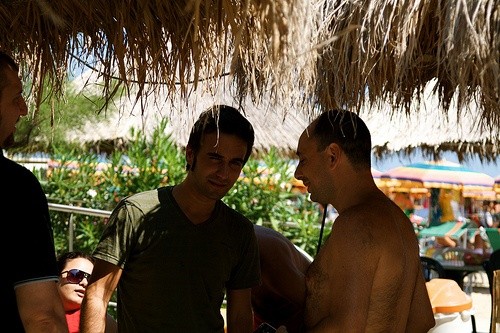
<box><xmin>425</xmin><ymin>277</ymin><xmax>477</xmax><ymax>333</ymax></box>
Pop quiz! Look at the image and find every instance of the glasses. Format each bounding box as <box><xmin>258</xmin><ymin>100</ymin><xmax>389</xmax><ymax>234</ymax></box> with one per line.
<box><xmin>61</xmin><ymin>269</ymin><xmax>91</xmax><ymax>285</ymax></box>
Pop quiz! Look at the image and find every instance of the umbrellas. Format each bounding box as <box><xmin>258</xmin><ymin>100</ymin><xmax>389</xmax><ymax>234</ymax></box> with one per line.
<box><xmin>369</xmin><ymin>157</ymin><xmax>500</xmax><ymax>225</ymax></box>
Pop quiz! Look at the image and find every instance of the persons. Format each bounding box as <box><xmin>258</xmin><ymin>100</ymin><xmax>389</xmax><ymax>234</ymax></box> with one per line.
<box><xmin>56</xmin><ymin>251</ymin><xmax>117</xmax><ymax>333</ymax></box>
<box><xmin>79</xmin><ymin>104</ymin><xmax>315</xmax><ymax>333</ymax></box>
<box><xmin>294</xmin><ymin>109</ymin><xmax>436</xmax><ymax>333</ymax></box>
<box><xmin>0</xmin><ymin>51</ymin><xmax>69</xmax><ymax>333</ymax></box>
